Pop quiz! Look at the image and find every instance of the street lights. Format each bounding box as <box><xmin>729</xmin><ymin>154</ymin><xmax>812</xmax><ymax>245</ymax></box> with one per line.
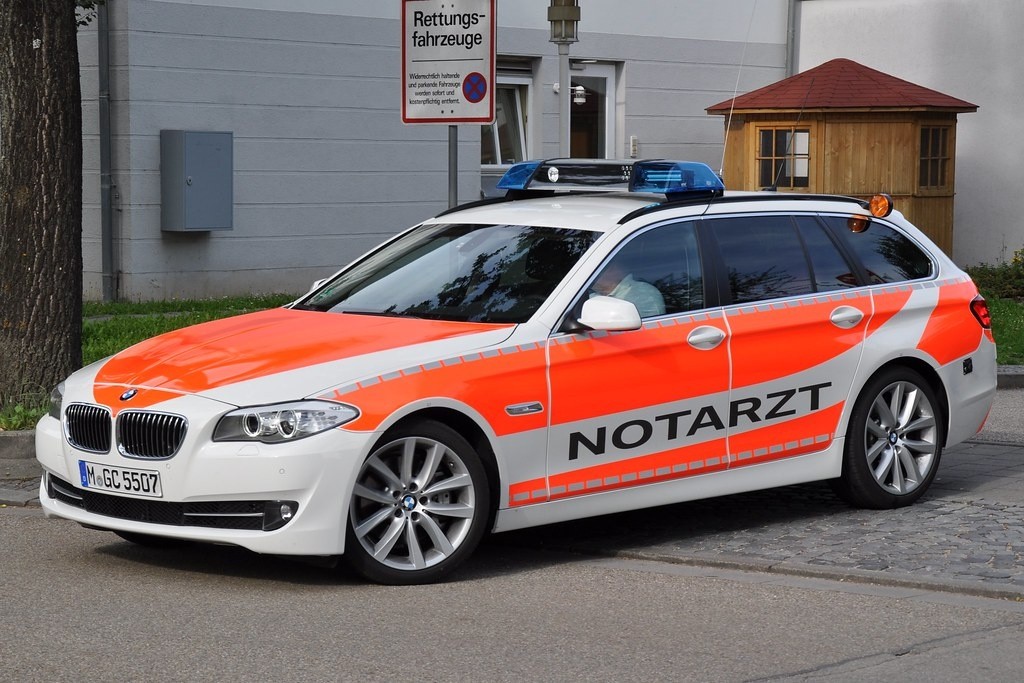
<box><xmin>548</xmin><ymin>0</ymin><xmax>581</xmax><ymax>159</ymax></box>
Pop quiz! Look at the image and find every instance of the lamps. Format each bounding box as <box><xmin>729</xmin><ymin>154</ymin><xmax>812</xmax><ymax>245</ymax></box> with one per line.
<box><xmin>553</xmin><ymin>82</ymin><xmax>586</xmax><ymax>105</ymax></box>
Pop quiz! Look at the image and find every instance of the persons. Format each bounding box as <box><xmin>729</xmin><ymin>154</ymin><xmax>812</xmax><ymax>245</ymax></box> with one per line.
<box><xmin>583</xmin><ymin>238</ymin><xmax>666</xmax><ymax>317</ymax></box>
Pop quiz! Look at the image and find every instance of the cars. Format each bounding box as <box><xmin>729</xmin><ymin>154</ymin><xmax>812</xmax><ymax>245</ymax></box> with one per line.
<box><xmin>34</xmin><ymin>157</ymin><xmax>997</xmax><ymax>586</ymax></box>
